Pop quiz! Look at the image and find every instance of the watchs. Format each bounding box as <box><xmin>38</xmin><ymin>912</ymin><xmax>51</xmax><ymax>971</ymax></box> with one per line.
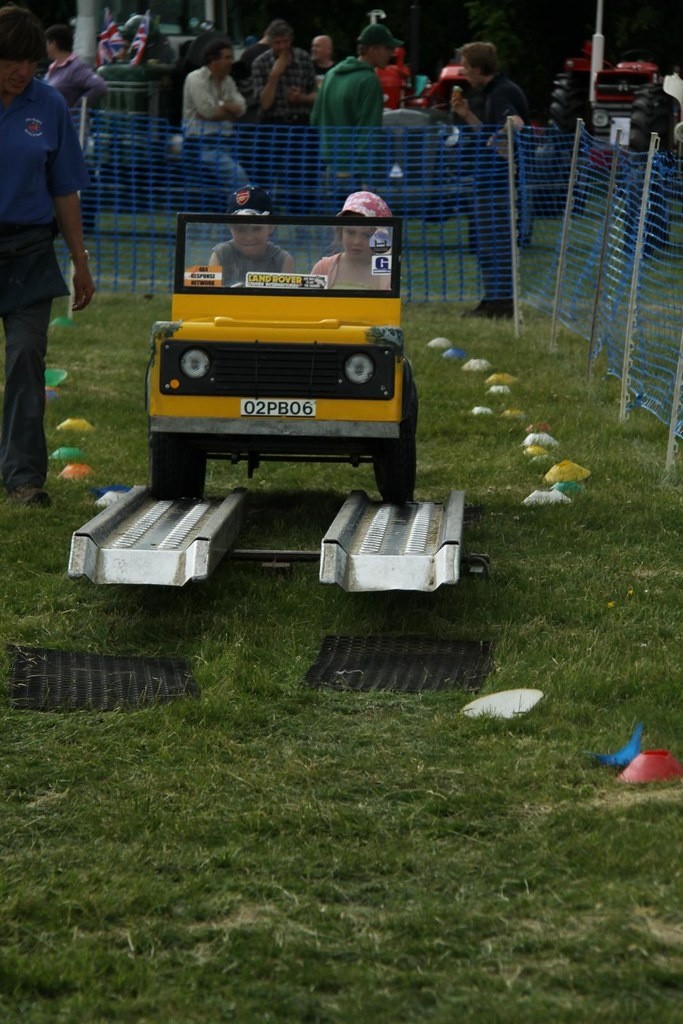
<box><xmin>69</xmin><ymin>250</ymin><xmax>90</xmax><ymax>261</ymax></box>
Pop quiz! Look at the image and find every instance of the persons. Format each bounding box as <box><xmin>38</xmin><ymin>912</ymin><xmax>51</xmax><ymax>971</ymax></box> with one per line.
<box><xmin>450</xmin><ymin>42</ymin><xmax>530</xmax><ymax>319</ymax></box>
<box><xmin>208</xmin><ymin>186</ymin><xmax>295</xmax><ymax>287</ymax></box>
<box><xmin>43</xmin><ymin>23</ymin><xmax>109</xmax><ymax>112</ymax></box>
<box><xmin>0</xmin><ymin>6</ymin><xmax>94</xmax><ymax>506</ymax></box>
<box><xmin>180</xmin><ymin>18</ymin><xmax>405</xmax><ymax>191</ymax></box>
<box><xmin>309</xmin><ymin>191</ymin><xmax>392</xmax><ymax>291</ymax></box>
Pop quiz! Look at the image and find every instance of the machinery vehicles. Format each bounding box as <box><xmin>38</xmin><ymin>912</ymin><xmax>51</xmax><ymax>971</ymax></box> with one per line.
<box><xmin>549</xmin><ymin>0</ymin><xmax>677</xmax><ymax>154</ymax></box>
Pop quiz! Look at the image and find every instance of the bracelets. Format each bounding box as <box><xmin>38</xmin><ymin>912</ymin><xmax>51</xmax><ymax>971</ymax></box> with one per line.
<box><xmin>218</xmin><ymin>100</ymin><xmax>224</xmax><ymax>108</ymax></box>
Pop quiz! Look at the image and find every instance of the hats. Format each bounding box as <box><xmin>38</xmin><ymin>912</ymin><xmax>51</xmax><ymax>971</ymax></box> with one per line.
<box><xmin>117</xmin><ymin>14</ymin><xmax>161</xmax><ymax>36</ymax></box>
<box><xmin>225</xmin><ymin>184</ymin><xmax>273</xmax><ymax>216</ymax></box>
<box><xmin>356</xmin><ymin>24</ymin><xmax>405</xmax><ymax>55</ymax></box>
<box><xmin>336</xmin><ymin>191</ymin><xmax>393</xmax><ymax>219</ymax></box>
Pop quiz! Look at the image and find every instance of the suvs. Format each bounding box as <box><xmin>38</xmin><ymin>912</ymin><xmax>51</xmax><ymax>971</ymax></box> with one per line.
<box><xmin>143</xmin><ymin>212</ymin><xmax>420</xmax><ymax>510</ymax></box>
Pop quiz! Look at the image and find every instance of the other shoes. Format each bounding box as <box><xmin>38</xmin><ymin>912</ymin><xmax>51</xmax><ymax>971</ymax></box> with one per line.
<box><xmin>496</xmin><ymin>299</ymin><xmax>513</xmax><ymax>320</ymax></box>
<box><xmin>4</xmin><ymin>485</ymin><xmax>52</xmax><ymax>507</ymax></box>
<box><xmin>460</xmin><ymin>300</ymin><xmax>496</xmax><ymax>320</ymax></box>
